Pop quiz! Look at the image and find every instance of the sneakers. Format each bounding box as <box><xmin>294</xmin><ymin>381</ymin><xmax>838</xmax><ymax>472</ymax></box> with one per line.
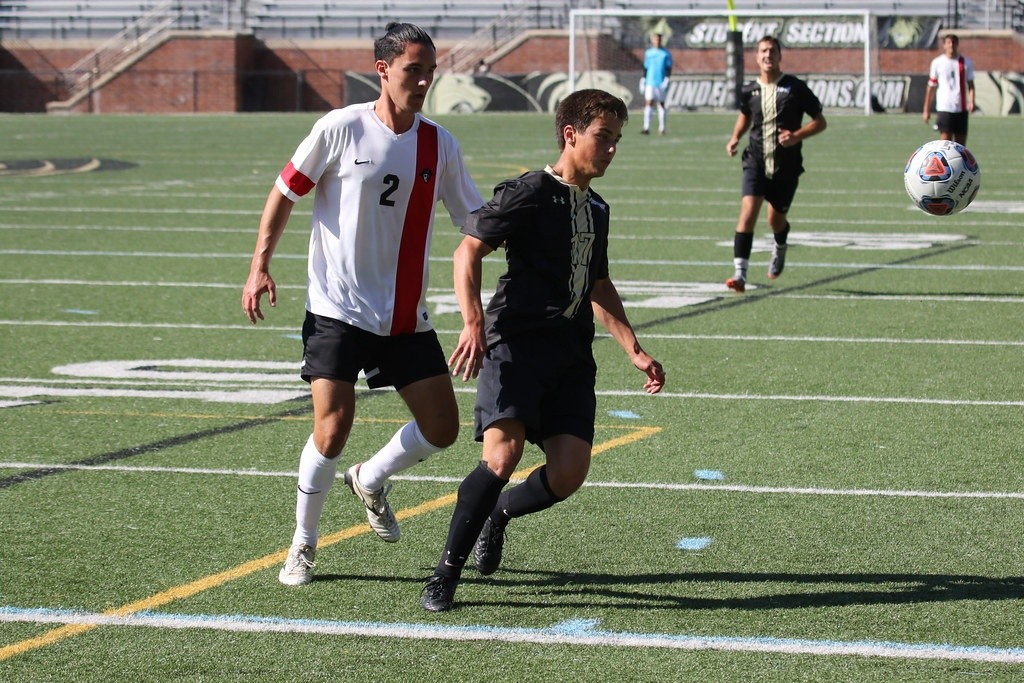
<box><xmin>768</xmin><ymin>255</ymin><xmax>785</xmax><ymax>278</ymax></box>
<box><xmin>278</xmin><ymin>540</ymin><xmax>317</xmax><ymax>585</ymax></box>
<box><xmin>727</xmin><ymin>275</ymin><xmax>745</xmax><ymax>292</ymax></box>
<box><xmin>475</xmin><ymin>516</ymin><xmax>508</xmax><ymax>576</ymax></box>
<box><xmin>420</xmin><ymin>573</ymin><xmax>461</xmax><ymax>611</ymax></box>
<box><xmin>344</xmin><ymin>464</ymin><xmax>400</xmax><ymax>543</ymax></box>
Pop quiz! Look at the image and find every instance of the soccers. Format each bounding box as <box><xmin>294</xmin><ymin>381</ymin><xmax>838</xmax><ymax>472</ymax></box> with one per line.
<box><xmin>903</xmin><ymin>140</ymin><xmax>981</xmax><ymax>216</ymax></box>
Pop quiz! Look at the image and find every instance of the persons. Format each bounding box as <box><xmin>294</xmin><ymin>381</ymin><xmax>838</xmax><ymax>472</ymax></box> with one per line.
<box><xmin>419</xmin><ymin>88</ymin><xmax>667</xmax><ymax>615</ymax></box>
<box><xmin>638</xmin><ymin>32</ymin><xmax>674</xmax><ymax>137</ymax></box>
<box><xmin>241</xmin><ymin>21</ymin><xmax>487</xmax><ymax>588</ymax></box>
<box><xmin>724</xmin><ymin>34</ymin><xmax>826</xmax><ymax>294</ymax></box>
<box><xmin>922</xmin><ymin>33</ymin><xmax>977</xmax><ymax>149</ymax></box>
